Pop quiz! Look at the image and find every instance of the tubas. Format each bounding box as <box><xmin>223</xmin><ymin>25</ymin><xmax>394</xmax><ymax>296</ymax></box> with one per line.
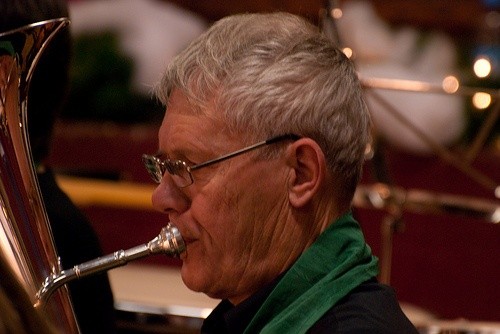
<box><xmin>0</xmin><ymin>16</ymin><xmax>187</xmax><ymax>333</ymax></box>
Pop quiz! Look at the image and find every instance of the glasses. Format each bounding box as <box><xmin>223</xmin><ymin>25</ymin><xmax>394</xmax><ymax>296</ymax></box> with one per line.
<box><xmin>141</xmin><ymin>132</ymin><xmax>303</xmax><ymax>188</ymax></box>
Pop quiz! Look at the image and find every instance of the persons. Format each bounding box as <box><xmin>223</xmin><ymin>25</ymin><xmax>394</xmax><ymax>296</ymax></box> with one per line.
<box><xmin>142</xmin><ymin>11</ymin><xmax>418</xmax><ymax>334</ymax></box>
<box><xmin>1</xmin><ymin>0</ymin><xmax>122</xmax><ymax>334</ymax></box>
